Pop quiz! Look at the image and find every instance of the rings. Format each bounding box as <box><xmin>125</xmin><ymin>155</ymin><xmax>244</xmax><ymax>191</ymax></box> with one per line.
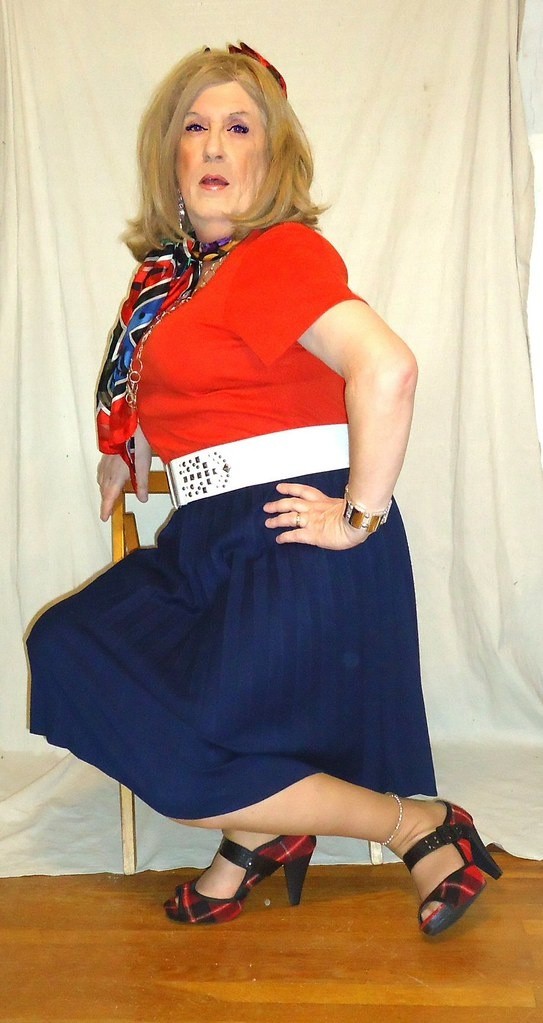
<box><xmin>296</xmin><ymin>513</ymin><xmax>300</xmax><ymax>528</ymax></box>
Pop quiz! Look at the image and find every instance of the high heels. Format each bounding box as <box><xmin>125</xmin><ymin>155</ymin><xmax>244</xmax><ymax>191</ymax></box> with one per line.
<box><xmin>163</xmin><ymin>832</ymin><xmax>317</xmax><ymax>924</ymax></box>
<box><xmin>403</xmin><ymin>796</ymin><xmax>504</xmax><ymax>935</ymax></box>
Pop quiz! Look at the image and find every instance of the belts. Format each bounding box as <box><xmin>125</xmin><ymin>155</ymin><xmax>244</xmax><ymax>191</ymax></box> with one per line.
<box><xmin>159</xmin><ymin>423</ymin><xmax>352</xmax><ymax>509</ymax></box>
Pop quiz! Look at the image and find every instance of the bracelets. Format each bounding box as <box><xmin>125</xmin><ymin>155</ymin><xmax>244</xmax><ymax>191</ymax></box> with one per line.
<box><xmin>343</xmin><ymin>484</ymin><xmax>393</xmax><ymax>533</ymax></box>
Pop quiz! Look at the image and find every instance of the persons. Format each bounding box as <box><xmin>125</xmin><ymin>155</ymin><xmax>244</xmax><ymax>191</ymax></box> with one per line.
<box><xmin>25</xmin><ymin>44</ymin><xmax>502</xmax><ymax>935</ymax></box>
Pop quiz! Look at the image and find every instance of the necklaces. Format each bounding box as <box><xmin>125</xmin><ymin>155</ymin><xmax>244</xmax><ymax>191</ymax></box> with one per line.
<box><xmin>126</xmin><ymin>252</ymin><xmax>230</xmax><ymax>409</ymax></box>
